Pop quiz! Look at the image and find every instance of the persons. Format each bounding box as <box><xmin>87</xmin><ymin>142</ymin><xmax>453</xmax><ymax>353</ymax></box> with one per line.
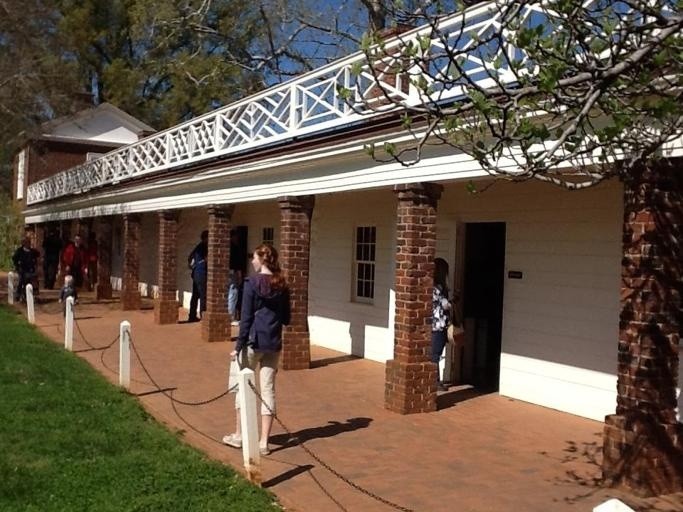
<box><xmin>432</xmin><ymin>258</ymin><xmax>460</xmax><ymax>387</ymax></box>
<box><xmin>188</xmin><ymin>230</ymin><xmax>244</xmax><ymax>326</ymax></box>
<box><xmin>12</xmin><ymin>230</ymin><xmax>98</xmax><ymax>317</ymax></box>
<box><xmin>222</xmin><ymin>244</ymin><xmax>290</xmax><ymax>455</ymax></box>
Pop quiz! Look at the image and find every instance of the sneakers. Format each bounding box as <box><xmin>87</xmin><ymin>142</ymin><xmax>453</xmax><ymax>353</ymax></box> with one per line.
<box><xmin>435</xmin><ymin>383</ymin><xmax>447</xmax><ymax>391</ymax></box>
<box><xmin>257</xmin><ymin>443</ymin><xmax>270</xmax><ymax>455</ymax></box>
<box><xmin>190</xmin><ymin>317</ymin><xmax>199</xmax><ymax>321</ymax></box>
<box><xmin>223</xmin><ymin>434</ymin><xmax>242</xmax><ymax>448</ymax></box>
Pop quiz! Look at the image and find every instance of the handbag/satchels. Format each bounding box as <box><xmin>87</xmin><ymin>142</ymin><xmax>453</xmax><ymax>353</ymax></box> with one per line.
<box><xmin>228</xmin><ymin>357</ymin><xmax>241</xmax><ymax>394</ymax></box>
<box><xmin>447</xmin><ymin>323</ymin><xmax>465</xmax><ymax>346</ymax></box>
